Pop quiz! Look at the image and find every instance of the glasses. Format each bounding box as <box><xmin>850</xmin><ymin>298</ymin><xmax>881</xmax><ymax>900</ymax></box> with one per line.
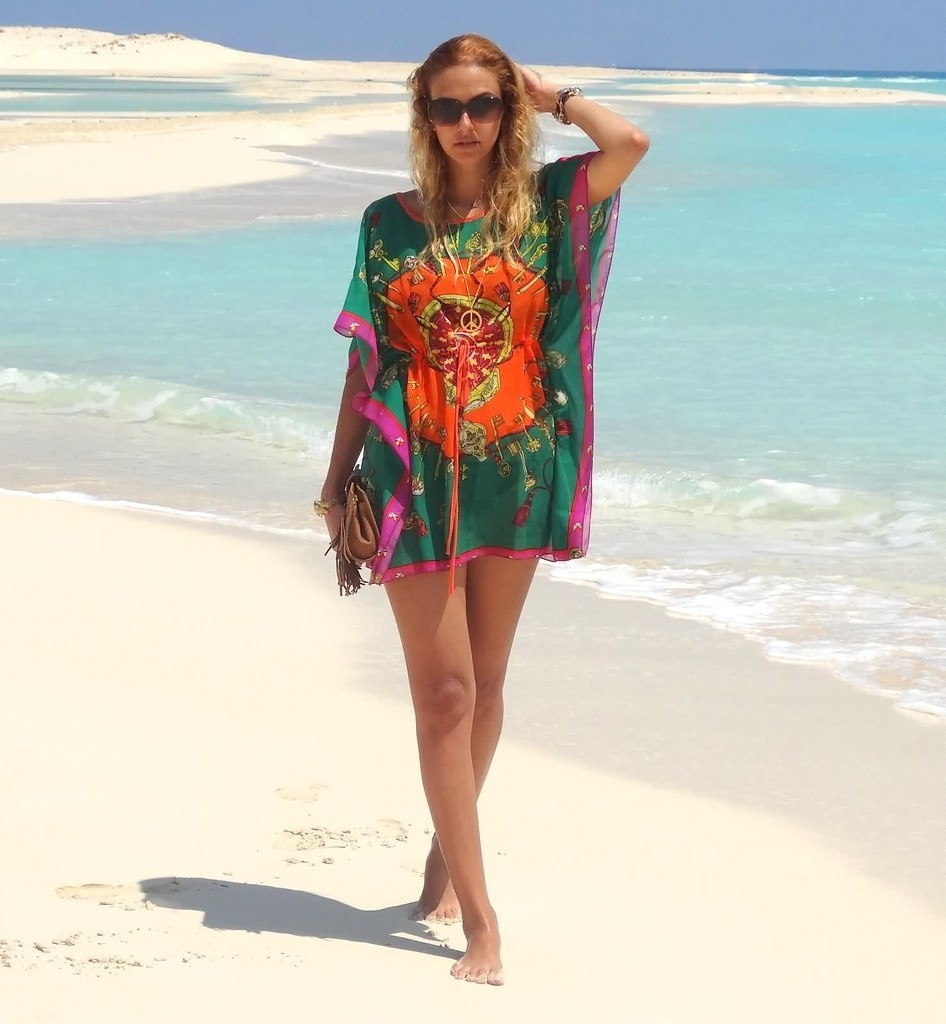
<box><xmin>423</xmin><ymin>92</ymin><xmax>508</xmax><ymax>126</ymax></box>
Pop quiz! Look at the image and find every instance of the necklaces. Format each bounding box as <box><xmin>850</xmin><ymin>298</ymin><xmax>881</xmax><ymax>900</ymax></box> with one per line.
<box><xmin>446</xmin><ymin>199</ymin><xmax>465</xmax><ymax>219</ymax></box>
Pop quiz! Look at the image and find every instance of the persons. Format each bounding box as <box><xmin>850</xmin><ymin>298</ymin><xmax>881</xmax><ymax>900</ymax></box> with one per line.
<box><xmin>321</xmin><ymin>33</ymin><xmax>651</xmax><ymax>987</ymax></box>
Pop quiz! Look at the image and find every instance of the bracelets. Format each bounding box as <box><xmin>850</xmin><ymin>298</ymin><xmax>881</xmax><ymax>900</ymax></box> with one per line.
<box><xmin>314</xmin><ymin>494</ymin><xmax>345</xmax><ymax>517</ymax></box>
<box><xmin>552</xmin><ymin>86</ymin><xmax>583</xmax><ymax>125</ymax></box>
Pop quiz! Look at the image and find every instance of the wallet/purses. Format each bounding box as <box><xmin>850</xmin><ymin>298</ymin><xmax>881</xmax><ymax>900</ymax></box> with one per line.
<box><xmin>324</xmin><ymin>463</ymin><xmax>381</xmax><ymax>597</ymax></box>
